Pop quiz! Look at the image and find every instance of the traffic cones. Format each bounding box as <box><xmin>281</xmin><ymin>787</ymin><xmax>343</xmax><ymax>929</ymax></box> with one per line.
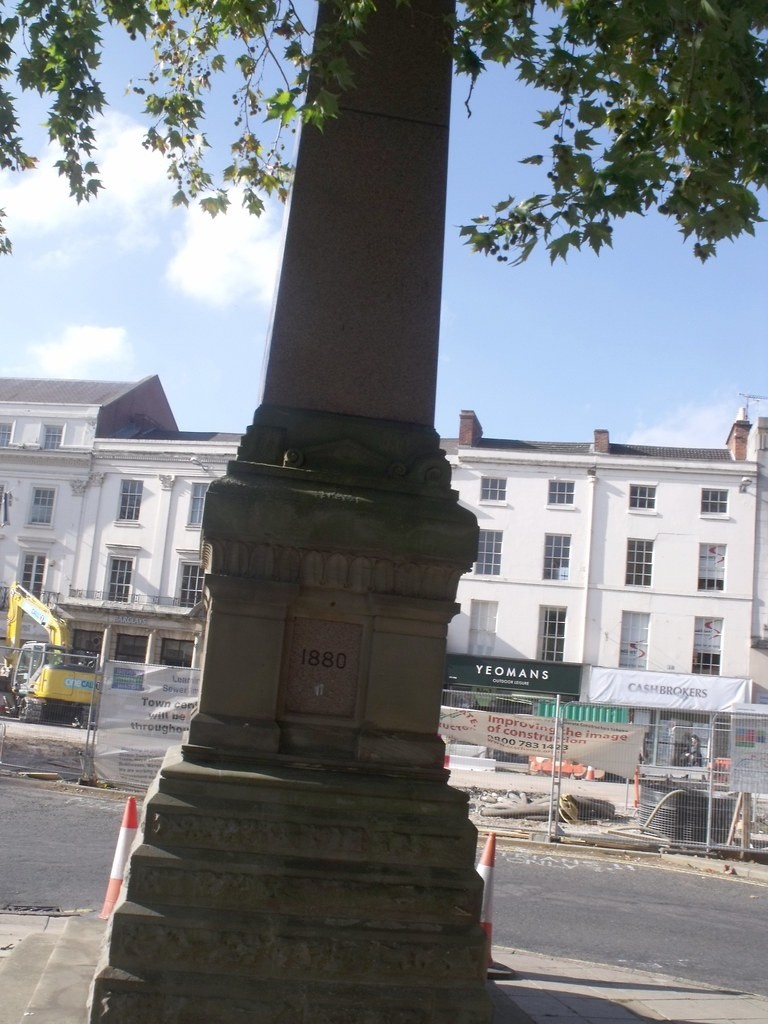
<box><xmin>97</xmin><ymin>795</ymin><xmax>139</xmax><ymax>921</ymax></box>
<box><xmin>581</xmin><ymin>765</ymin><xmax>599</xmax><ymax>783</ymax></box>
<box><xmin>474</xmin><ymin>830</ymin><xmax>511</xmax><ymax>979</ymax></box>
<box><xmin>439</xmin><ymin>732</ymin><xmax>451</xmax><ymax>769</ymax></box>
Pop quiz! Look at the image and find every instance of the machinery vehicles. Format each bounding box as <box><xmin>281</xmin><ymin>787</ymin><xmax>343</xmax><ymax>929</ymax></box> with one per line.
<box><xmin>1</xmin><ymin>581</ymin><xmax>107</xmax><ymax>725</ymax></box>
